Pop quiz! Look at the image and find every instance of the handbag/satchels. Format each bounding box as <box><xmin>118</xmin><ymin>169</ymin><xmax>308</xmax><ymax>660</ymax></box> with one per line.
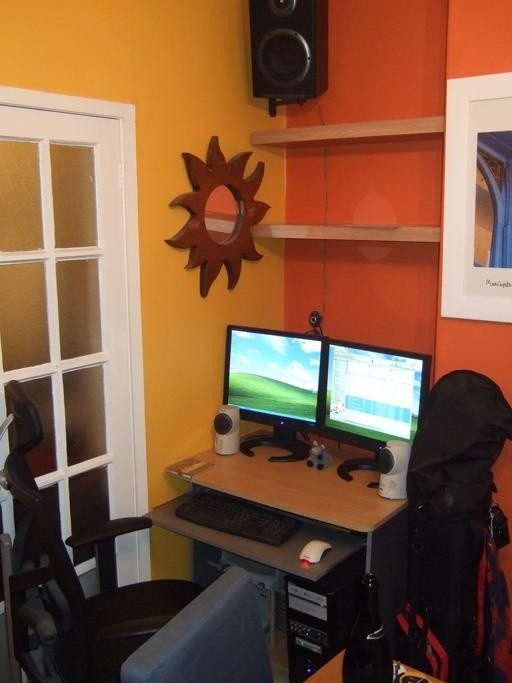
<box><xmin>407</xmin><ymin>370</ymin><xmax>512</xmax><ymax>511</ymax></box>
<box><xmin>395</xmin><ymin>511</ymin><xmax>509</xmax><ymax>682</ymax></box>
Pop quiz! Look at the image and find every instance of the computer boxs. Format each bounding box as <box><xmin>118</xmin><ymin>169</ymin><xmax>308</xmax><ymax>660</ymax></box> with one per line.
<box><xmin>284</xmin><ymin>555</ymin><xmax>366</xmax><ymax>683</ymax></box>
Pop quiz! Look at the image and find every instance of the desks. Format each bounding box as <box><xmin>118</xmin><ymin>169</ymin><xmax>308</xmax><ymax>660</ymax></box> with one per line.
<box><xmin>141</xmin><ymin>429</ymin><xmax>409</xmax><ymax>670</ymax></box>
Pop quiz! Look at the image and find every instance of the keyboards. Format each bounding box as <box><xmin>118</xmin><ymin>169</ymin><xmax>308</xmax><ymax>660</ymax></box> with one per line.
<box><xmin>175</xmin><ymin>492</ymin><xmax>301</xmax><ymax>547</ymax></box>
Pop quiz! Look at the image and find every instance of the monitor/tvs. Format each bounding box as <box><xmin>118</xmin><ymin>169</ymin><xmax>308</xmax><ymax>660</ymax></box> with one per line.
<box><xmin>223</xmin><ymin>324</ymin><xmax>330</xmax><ymax>463</ymax></box>
<box><xmin>317</xmin><ymin>338</ymin><xmax>433</xmax><ymax>489</ymax></box>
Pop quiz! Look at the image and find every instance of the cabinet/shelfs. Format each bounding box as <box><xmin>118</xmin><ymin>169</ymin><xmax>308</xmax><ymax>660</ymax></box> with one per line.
<box><xmin>248</xmin><ymin>116</ymin><xmax>445</xmax><ymax>244</ymax></box>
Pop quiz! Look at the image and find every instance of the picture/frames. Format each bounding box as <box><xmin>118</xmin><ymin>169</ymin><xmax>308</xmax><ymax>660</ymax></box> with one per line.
<box><xmin>439</xmin><ymin>70</ymin><xmax>512</xmax><ymax>324</ymax></box>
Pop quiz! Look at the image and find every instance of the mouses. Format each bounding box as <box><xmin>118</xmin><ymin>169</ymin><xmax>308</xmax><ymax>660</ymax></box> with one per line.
<box><xmin>298</xmin><ymin>540</ymin><xmax>332</xmax><ymax>564</ymax></box>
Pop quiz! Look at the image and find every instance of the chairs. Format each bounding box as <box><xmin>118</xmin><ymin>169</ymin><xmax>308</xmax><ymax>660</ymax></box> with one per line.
<box><xmin>1</xmin><ymin>379</ymin><xmax>203</xmax><ymax>680</ymax></box>
<box><xmin>120</xmin><ymin>564</ymin><xmax>273</xmax><ymax>682</ymax></box>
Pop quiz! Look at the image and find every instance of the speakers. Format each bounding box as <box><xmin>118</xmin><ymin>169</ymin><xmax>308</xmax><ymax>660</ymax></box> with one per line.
<box><xmin>213</xmin><ymin>405</ymin><xmax>240</xmax><ymax>457</ymax></box>
<box><xmin>377</xmin><ymin>440</ymin><xmax>412</xmax><ymax>500</ymax></box>
<box><xmin>248</xmin><ymin>0</ymin><xmax>329</xmax><ymax>100</ymax></box>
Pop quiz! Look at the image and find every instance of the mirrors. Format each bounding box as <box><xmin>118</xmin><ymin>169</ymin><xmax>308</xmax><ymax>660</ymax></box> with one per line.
<box><xmin>163</xmin><ymin>134</ymin><xmax>273</xmax><ymax>297</ymax></box>
